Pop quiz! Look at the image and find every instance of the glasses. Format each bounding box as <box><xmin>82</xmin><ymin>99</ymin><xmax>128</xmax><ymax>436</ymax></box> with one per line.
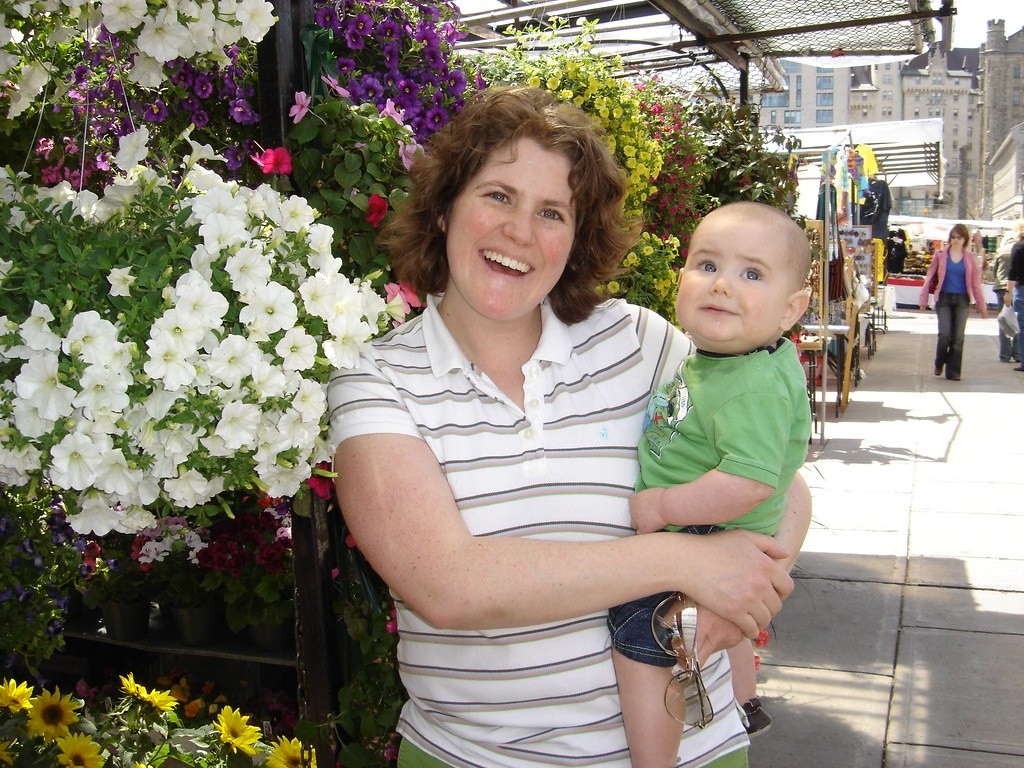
<box><xmin>651</xmin><ymin>591</ymin><xmax>714</xmax><ymax>729</ymax></box>
<box><xmin>951</xmin><ymin>236</ymin><xmax>961</xmax><ymax>240</ymax></box>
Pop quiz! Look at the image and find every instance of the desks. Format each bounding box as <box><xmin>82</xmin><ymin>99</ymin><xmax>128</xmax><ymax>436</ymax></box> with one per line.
<box><xmin>886</xmin><ymin>284</ymin><xmax>936</xmax><ymax>311</ymax></box>
<box><xmin>801</xmin><ymin>324</ymin><xmax>850</xmax><ymax>418</ymax></box>
<box><xmin>799</xmin><ymin>336</ymin><xmax>833</xmax><ymax>444</ymax></box>
<box><xmin>971</xmin><ymin>284</ymin><xmax>998</xmax><ymax>313</ymax></box>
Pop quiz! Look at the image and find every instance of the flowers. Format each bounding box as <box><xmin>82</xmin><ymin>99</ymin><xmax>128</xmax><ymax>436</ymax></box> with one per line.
<box><xmin>0</xmin><ymin>0</ymin><xmax>805</xmax><ymax>768</ymax></box>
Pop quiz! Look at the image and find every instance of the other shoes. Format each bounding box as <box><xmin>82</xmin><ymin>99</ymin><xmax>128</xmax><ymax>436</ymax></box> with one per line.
<box><xmin>1014</xmin><ymin>367</ymin><xmax>1024</xmax><ymax>372</ymax></box>
<box><xmin>935</xmin><ymin>368</ymin><xmax>942</xmax><ymax>375</ymax></box>
<box><xmin>947</xmin><ymin>376</ymin><xmax>960</xmax><ymax>380</ymax></box>
<box><xmin>742</xmin><ymin>698</ymin><xmax>771</xmax><ymax>738</ymax></box>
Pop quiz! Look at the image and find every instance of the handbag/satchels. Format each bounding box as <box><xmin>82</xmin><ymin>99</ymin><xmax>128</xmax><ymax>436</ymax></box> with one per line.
<box><xmin>998</xmin><ymin>303</ymin><xmax>1021</xmax><ymax>337</ymax></box>
<box><xmin>928</xmin><ymin>266</ymin><xmax>938</xmax><ymax>293</ymax></box>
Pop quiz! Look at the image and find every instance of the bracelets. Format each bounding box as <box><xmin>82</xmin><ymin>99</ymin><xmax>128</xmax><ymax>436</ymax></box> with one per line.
<box><xmin>1005</xmin><ymin>290</ymin><xmax>1012</xmax><ymax>293</ymax></box>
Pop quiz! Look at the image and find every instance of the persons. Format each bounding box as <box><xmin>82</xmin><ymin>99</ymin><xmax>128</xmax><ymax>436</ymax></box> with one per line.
<box><xmin>326</xmin><ymin>89</ymin><xmax>811</xmax><ymax>768</ymax></box>
<box><xmin>993</xmin><ymin>223</ymin><xmax>1024</xmax><ymax>371</ymax></box>
<box><xmin>919</xmin><ymin>224</ymin><xmax>989</xmax><ymax>380</ymax></box>
